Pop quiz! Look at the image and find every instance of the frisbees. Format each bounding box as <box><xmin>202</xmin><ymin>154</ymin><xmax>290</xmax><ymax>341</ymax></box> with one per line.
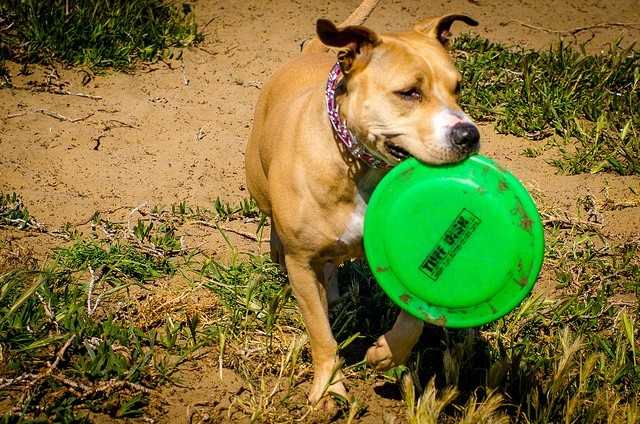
<box><xmin>363</xmin><ymin>154</ymin><xmax>545</xmax><ymax>329</ymax></box>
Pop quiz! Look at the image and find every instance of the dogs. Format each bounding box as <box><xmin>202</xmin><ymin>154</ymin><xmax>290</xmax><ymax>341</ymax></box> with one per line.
<box><xmin>244</xmin><ymin>0</ymin><xmax>480</xmax><ymax>408</ymax></box>
